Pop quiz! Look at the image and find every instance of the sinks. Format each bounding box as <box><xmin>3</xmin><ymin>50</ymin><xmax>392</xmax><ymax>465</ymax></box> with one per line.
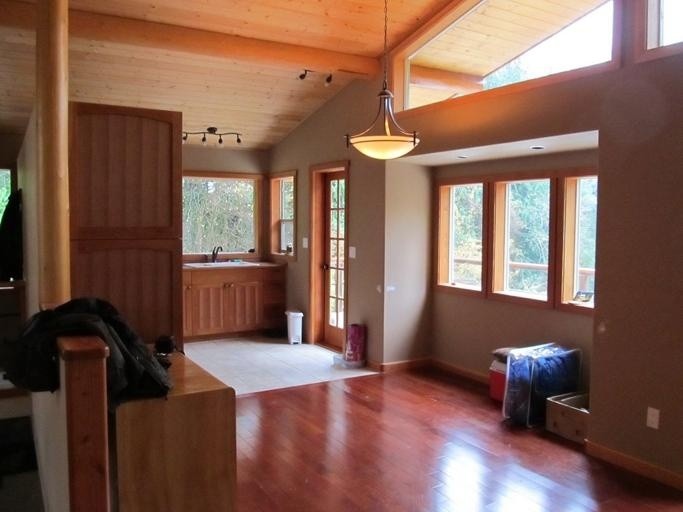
<box><xmin>184</xmin><ymin>262</ymin><xmax>259</xmax><ymax>268</ymax></box>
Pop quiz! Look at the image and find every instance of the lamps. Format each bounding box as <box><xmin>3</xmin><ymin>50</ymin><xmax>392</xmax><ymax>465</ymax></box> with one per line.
<box><xmin>343</xmin><ymin>0</ymin><xmax>422</xmax><ymax>162</ymax></box>
<box><xmin>298</xmin><ymin>69</ymin><xmax>333</xmax><ymax>88</ymax></box>
<box><xmin>181</xmin><ymin>127</ymin><xmax>242</xmax><ymax>147</ymax></box>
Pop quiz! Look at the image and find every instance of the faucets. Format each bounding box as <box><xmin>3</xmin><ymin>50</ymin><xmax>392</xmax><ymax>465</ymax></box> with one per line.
<box><xmin>213</xmin><ymin>246</ymin><xmax>223</xmax><ymax>263</ymax></box>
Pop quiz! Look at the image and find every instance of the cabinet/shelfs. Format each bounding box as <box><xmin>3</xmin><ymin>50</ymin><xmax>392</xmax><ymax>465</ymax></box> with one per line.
<box><xmin>182</xmin><ymin>271</ymin><xmax>288</xmax><ymax>339</ymax></box>
<box><xmin>116</xmin><ymin>341</ymin><xmax>237</xmax><ymax>511</ymax></box>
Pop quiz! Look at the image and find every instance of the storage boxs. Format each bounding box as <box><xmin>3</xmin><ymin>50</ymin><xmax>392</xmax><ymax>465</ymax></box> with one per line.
<box><xmin>487</xmin><ymin>360</ymin><xmax>508</xmax><ymax>402</ymax></box>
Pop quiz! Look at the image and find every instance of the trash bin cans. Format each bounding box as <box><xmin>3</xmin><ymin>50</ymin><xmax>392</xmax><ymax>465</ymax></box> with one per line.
<box><xmin>343</xmin><ymin>323</ymin><xmax>366</xmax><ymax>368</ymax></box>
<box><xmin>284</xmin><ymin>310</ymin><xmax>305</xmax><ymax>345</ymax></box>
